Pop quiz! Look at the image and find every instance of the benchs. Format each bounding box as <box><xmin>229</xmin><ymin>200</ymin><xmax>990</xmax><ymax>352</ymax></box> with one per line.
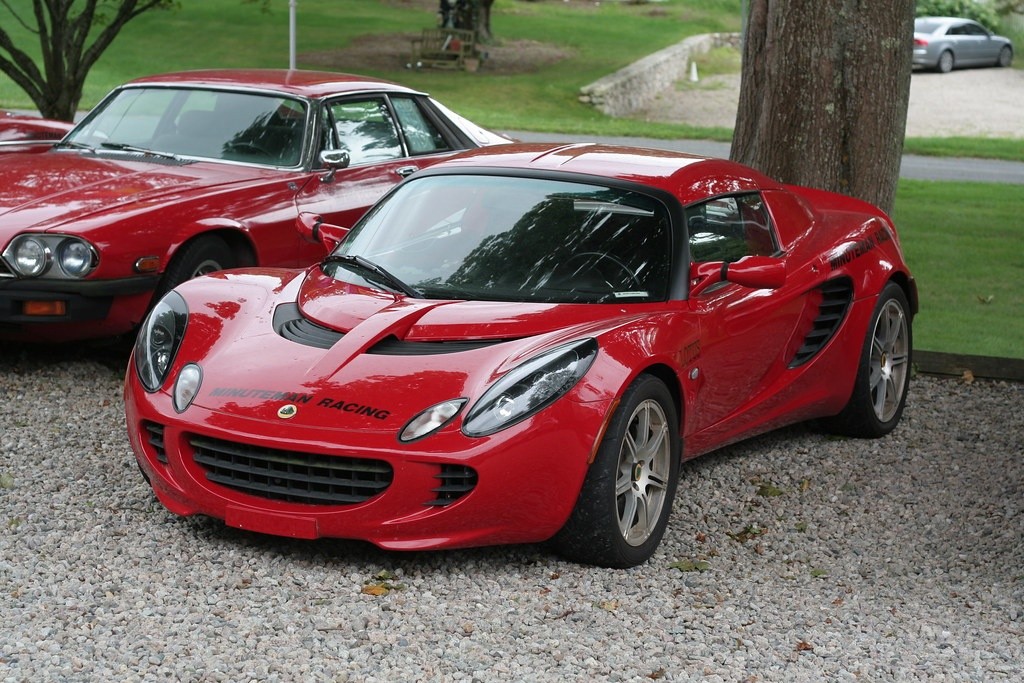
<box><xmin>410</xmin><ymin>24</ymin><xmax>477</xmax><ymax>70</ymax></box>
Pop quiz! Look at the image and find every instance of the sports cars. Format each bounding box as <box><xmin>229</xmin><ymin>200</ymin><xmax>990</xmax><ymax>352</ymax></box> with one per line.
<box><xmin>0</xmin><ymin>66</ymin><xmax>523</xmax><ymax>340</ymax></box>
<box><xmin>121</xmin><ymin>141</ymin><xmax>918</xmax><ymax>570</ymax></box>
<box><xmin>0</xmin><ymin>105</ymin><xmax>108</xmax><ymax>152</ymax></box>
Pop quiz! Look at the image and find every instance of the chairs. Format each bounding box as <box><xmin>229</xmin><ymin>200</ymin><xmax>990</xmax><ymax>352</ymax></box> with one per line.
<box><xmin>227</xmin><ymin>118</ymin><xmax>302</xmax><ymax>170</ymax></box>
<box><xmin>157</xmin><ymin>108</ymin><xmax>234</xmax><ymax>160</ymax></box>
<box><xmin>441</xmin><ymin>192</ymin><xmax>577</xmax><ymax>292</ymax></box>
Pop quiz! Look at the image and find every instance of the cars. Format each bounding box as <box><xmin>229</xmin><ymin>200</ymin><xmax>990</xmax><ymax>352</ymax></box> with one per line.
<box><xmin>912</xmin><ymin>14</ymin><xmax>1015</xmax><ymax>74</ymax></box>
